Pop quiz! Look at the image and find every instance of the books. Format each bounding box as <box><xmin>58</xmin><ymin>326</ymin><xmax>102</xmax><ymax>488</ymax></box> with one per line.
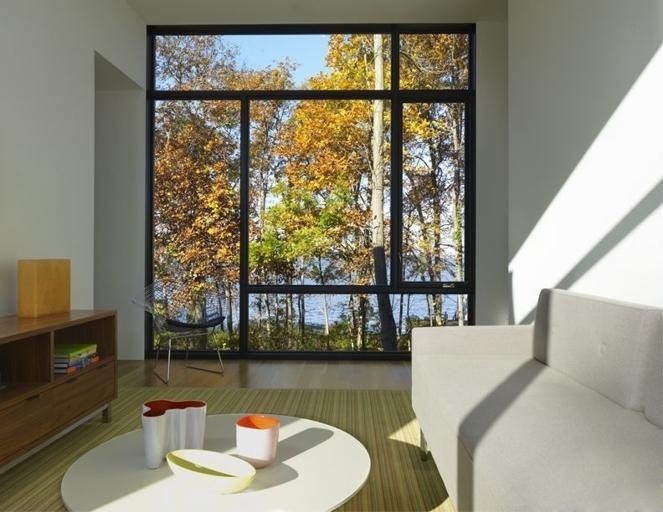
<box><xmin>54</xmin><ymin>341</ymin><xmax>98</xmax><ymax>373</ymax></box>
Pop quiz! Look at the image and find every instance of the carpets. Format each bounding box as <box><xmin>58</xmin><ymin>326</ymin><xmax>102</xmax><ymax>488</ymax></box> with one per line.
<box><xmin>0</xmin><ymin>387</ymin><xmax>456</xmax><ymax>512</ymax></box>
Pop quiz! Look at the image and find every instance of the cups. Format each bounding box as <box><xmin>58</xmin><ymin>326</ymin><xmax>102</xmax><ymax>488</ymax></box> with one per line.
<box><xmin>235</xmin><ymin>414</ymin><xmax>280</xmax><ymax>469</ymax></box>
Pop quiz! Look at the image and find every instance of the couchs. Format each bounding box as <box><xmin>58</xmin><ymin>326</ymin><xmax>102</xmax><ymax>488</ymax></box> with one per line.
<box><xmin>411</xmin><ymin>288</ymin><xmax>662</xmax><ymax>512</ymax></box>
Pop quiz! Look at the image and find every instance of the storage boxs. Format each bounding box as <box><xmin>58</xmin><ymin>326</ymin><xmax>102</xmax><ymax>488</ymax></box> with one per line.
<box><xmin>18</xmin><ymin>258</ymin><xmax>70</xmax><ymax>318</ymax></box>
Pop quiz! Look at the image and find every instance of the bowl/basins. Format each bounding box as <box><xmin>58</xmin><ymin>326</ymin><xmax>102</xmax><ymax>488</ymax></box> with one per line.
<box><xmin>165</xmin><ymin>448</ymin><xmax>257</xmax><ymax>494</ymax></box>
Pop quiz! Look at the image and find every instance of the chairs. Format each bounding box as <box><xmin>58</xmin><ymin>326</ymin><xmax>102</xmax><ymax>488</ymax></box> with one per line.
<box><xmin>130</xmin><ymin>279</ymin><xmax>232</xmax><ymax>386</ymax></box>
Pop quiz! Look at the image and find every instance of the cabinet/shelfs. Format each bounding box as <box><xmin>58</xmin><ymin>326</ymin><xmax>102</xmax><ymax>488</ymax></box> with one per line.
<box><xmin>0</xmin><ymin>310</ymin><xmax>118</xmax><ymax>473</ymax></box>
